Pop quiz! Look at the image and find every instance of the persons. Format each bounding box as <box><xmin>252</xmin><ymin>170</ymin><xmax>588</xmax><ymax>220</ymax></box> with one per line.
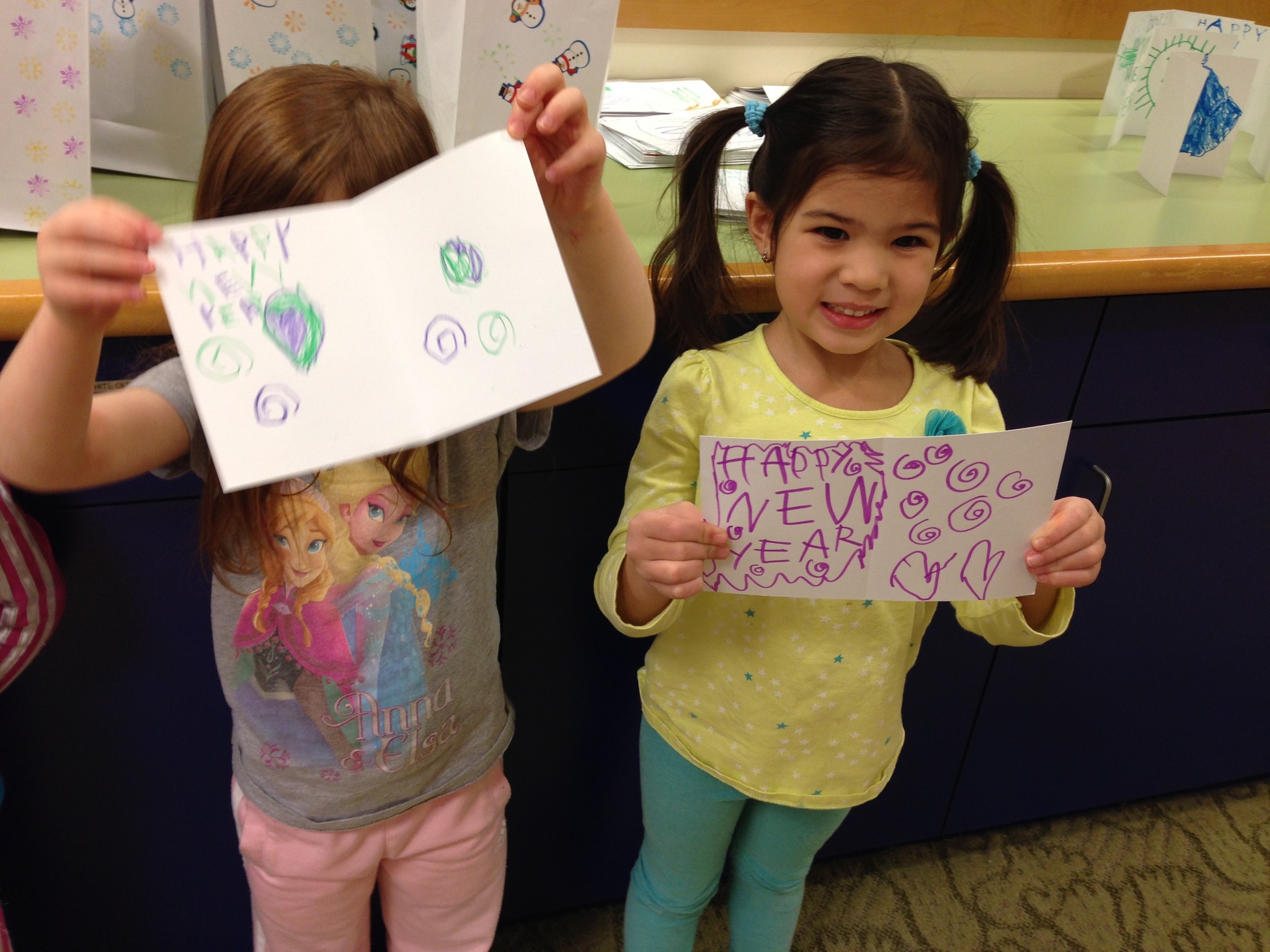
<box><xmin>0</xmin><ymin>59</ymin><xmax>659</xmax><ymax>952</ymax></box>
<box><xmin>591</xmin><ymin>54</ymin><xmax>1110</xmax><ymax>951</ymax></box>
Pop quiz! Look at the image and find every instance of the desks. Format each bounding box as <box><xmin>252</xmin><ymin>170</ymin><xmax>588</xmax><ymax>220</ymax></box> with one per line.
<box><xmin>1</xmin><ymin>86</ymin><xmax>1270</xmax><ymax>950</ymax></box>
